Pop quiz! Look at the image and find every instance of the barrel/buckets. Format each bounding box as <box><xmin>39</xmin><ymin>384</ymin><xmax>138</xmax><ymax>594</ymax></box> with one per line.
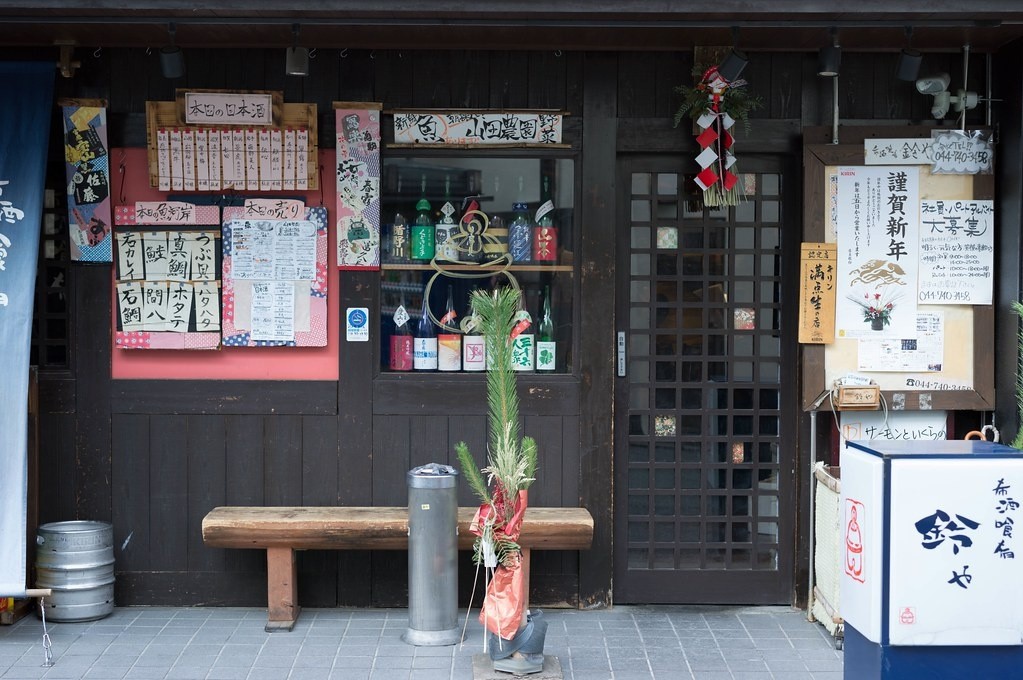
<box><xmin>33</xmin><ymin>518</ymin><xmax>114</xmax><ymax>623</ymax></box>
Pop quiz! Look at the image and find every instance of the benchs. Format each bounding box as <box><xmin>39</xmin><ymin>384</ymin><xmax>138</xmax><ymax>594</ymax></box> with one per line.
<box><xmin>202</xmin><ymin>506</ymin><xmax>595</xmax><ymax>632</ymax></box>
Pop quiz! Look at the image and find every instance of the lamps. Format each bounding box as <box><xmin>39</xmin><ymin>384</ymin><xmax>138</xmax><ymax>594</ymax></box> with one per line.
<box><xmin>286</xmin><ymin>22</ymin><xmax>309</xmax><ymax>76</ymax></box>
<box><xmin>716</xmin><ymin>30</ymin><xmax>759</xmax><ymax>82</ymax></box>
<box><xmin>817</xmin><ymin>26</ymin><xmax>844</xmax><ymax>77</ymax></box>
<box><xmin>915</xmin><ymin>52</ymin><xmax>981</xmax><ymax>121</ymax></box>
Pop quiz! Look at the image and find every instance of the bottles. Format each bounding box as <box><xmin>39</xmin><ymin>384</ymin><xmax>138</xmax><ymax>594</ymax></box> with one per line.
<box><xmin>434</xmin><ymin>173</ymin><xmax>460</xmax><ymax>264</ymax></box>
<box><xmin>391</xmin><ymin>173</ymin><xmax>412</xmax><ymax>263</ymax></box>
<box><xmin>410</xmin><ymin>171</ymin><xmax>436</xmax><ymax>264</ymax></box>
<box><xmin>438</xmin><ymin>284</ymin><xmax>462</xmax><ymax>373</ymax></box>
<box><xmin>459</xmin><ymin>174</ymin><xmax>484</xmax><ymax>265</ymax></box>
<box><xmin>462</xmin><ymin>284</ymin><xmax>487</xmax><ymax>373</ymax></box>
<box><xmin>535</xmin><ymin>284</ymin><xmax>558</xmax><ymax>372</ymax></box>
<box><xmin>481</xmin><ymin>173</ymin><xmax>509</xmax><ymax>265</ymax></box>
<box><xmin>508</xmin><ymin>285</ymin><xmax>535</xmax><ymax>372</ymax></box>
<box><xmin>491</xmin><ymin>288</ymin><xmax>501</xmax><ymax>302</ymax></box>
<box><xmin>508</xmin><ymin>175</ymin><xmax>533</xmax><ymax>265</ymax></box>
<box><xmin>413</xmin><ymin>297</ymin><xmax>439</xmax><ymax>372</ymax></box>
<box><xmin>532</xmin><ymin>174</ymin><xmax>562</xmax><ymax>265</ymax></box>
<box><xmin>388</xmin><ymin>293</ymin><xmax>414</xmax><ymax>372</ymax></box>
